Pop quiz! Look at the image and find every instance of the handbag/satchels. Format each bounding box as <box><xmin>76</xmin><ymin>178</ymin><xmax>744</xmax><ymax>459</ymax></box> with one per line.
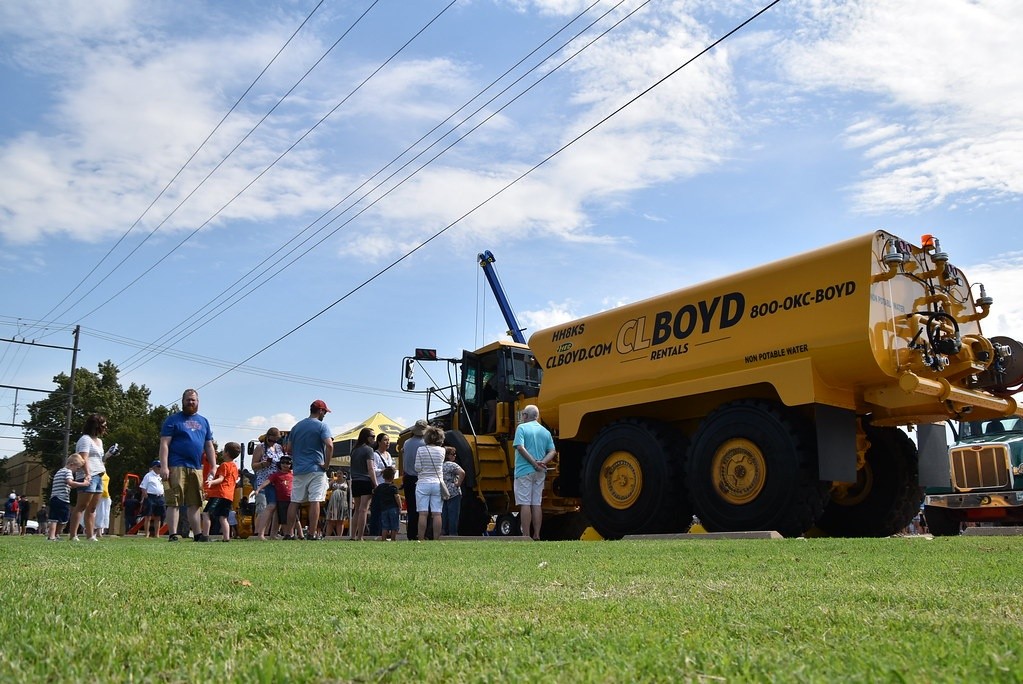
<box><xmin>440</xmin><ymin>482</ymin><xmax>450</xmax><ymax>500</ymax></box>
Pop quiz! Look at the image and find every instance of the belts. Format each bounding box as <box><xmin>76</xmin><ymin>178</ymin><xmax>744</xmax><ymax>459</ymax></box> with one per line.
<box><xmin>147</xmin><ymin>494</ymin><xmax>164</xmax><ymax>497</ymax></box>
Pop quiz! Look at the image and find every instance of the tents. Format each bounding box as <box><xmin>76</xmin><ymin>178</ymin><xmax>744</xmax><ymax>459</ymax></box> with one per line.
<box><xmin>326</xmin><ymin>412</ymin><xmax>407</xmax><ymax>535</ymax></box>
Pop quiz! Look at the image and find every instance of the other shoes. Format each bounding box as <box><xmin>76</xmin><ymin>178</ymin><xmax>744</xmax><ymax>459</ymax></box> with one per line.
<box><xmin>87</xmin><ymin>535</ymin><xmax>99</xmax><ymax>541</ymax></box>
<box><xmin>298</xmin><ymin>537</ymin><xmax>305</xmax><ymax>540</ymax></box>
<box><xmin>222</xmin><ymin>539</ymin><xmax>230</xmax><ymax>542</ymax></box>
<box><xmin>307</xmin><ymin>534</ymin><xmax>321</xmax><ymax>541</ymax></box>
<box><xmin>230</xmin><ymin>536</ymin><xmax>237</xmax><ymax>539</ymax></box>
<box><xmin>46</xmin><ymin>537</ymin><xmax>63</xmax><ymax>541</ymax></box>
<box><xmin>533</xmin><ymin>539</ymin><xmax>540</xmax><ymax>541</ymax></box>
<box><xmin>283</xmin><ymin>534</ymin><xmax>297</xmax><ymax>540</ymax></box>
<box><xmin>70</xmin><ymin>536</ymin><xmax>81</xmax><ymax>542</ymax></box>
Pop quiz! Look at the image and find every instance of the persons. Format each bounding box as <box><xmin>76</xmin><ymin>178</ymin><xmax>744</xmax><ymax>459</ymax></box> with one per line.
<box><xmin>402</xmin><ymin>419</ymin><xmax>465</xmax><ymax>541</ymax></box>
<box><xmin>36</xmin><ymin>413</ymin><xmax>118</xmax><ymax>542</ymax></box>
<box><xmin>252</xmin><ymin>428</ymin><xmax>402</xmax><ymax>542</ymax></box>
<box><xmin>482</xmin><ymin>369</ymin><xmax>510</xmax><ymax>434</ymax></box>
<box><xmin>159</xmin><ymin>389</ymin><xmax>217</xmax><ymax>542</ymax></box>
<box><xmin>282</xmin><ymin>400</ymin><xmax>334</xmax><ymax>541</ymax></box>
<box><xmin>0</xmin><ymin>493</ymin><xmax>30</xmax><ymax>536</ymax></box>
<box><xmin>513</xmin><ymin>405</ymin><xmax>557</xmax><ymax>541</ymax></box>
<box><xmin>122</xmin><ymin>441</ymin><xmax>241</xmax><ymax>542</ymax></box>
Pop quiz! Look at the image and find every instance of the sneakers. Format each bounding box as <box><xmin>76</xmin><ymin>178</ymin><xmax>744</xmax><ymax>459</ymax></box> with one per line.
<box><xmin>194</xmin><ymin>533</ymin><xmax>210</xmax><ymax>542</ymax></box>
<box><xmin>168</xmin><ymin>533</ymin><xmax>178</xmax><ymax>541</ymax></box>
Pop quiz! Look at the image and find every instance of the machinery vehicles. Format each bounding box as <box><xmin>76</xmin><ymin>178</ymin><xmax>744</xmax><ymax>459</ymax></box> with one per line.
<box><xmin>122</xmin><ymin>474</ymin><xmax>193</xmax><ymax>539</ymax></box>
<box><xmin>400</xmin><ymin>230</ymin><xmax>1023</xmax><ymax>540</ymax></box>
<box><xmin>228</xmin><ymin>472</ymin><xmax>355</xmax><ymax>538</ymax></box>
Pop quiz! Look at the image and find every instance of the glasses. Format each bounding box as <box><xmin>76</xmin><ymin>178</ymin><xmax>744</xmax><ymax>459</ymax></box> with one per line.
<box><xmin>102</xmin><ymin>424</ymin><xmax>107</xmax><ymax>428</ymax></box>
<box><xmin>368</xmin><ymin>435</ymin><xmax>375</xmax><ymax>438</ymax></box>
<box><xmin>448</xmin><ymin>452</ymin><xmax>456</xmax><ymax>457</ymax></box>
<box><xmin>522</xmin><ymin>412</ymin><xmax>526</xmax><ymax>416</ymax></box>
<box><xmin>268</xmin><ymin>435</ymin><xmax>278</xmax><ymax>442</ymax></box>
<box><xmin>280</xmin><ymin>460</ymin><xmax>291</xmax><ymax>464</ymax></box>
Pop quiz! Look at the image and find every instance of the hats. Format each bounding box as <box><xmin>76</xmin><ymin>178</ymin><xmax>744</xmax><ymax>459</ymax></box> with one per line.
<box><xmin>413</xmin><ymin>420</ymin><xmax>427</xmax><ymax>435</ymax></box>
<box><xmin>152</xmin><ymin>460</ymin><xmax>161</xmax><ymax>466</ymax></box>
<box><xmin>20</xmin><ymin>495</ymin><xmax>26</xmax><ymax>498</ymax></box>
<box><xmin>310</xmin><ymin>400</ymin><xmax>331</xmax><ymax>413</ymax></box>
<box><xmin>280</xmin><ymin>454</ymin><xmax>292</xmax><ymax>460</ymax></box>
<box><xmin>10</xmin><ymin>493</ymin><xmax>16</xmax><ymax>499</ymax></box>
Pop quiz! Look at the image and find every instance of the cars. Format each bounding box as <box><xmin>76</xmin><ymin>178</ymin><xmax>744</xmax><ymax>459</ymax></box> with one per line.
<box><xmin>0</xmin><ymin>510</ymin><xmax>39</xmax><ymax>536</ymax></box>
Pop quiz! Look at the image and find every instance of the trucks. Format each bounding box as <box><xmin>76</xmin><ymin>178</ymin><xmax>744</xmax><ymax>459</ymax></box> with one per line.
<box><xmin>907</xmin><ymin>414</ymin><xmax>1023</xmax><ymax>536</ymax></box>
